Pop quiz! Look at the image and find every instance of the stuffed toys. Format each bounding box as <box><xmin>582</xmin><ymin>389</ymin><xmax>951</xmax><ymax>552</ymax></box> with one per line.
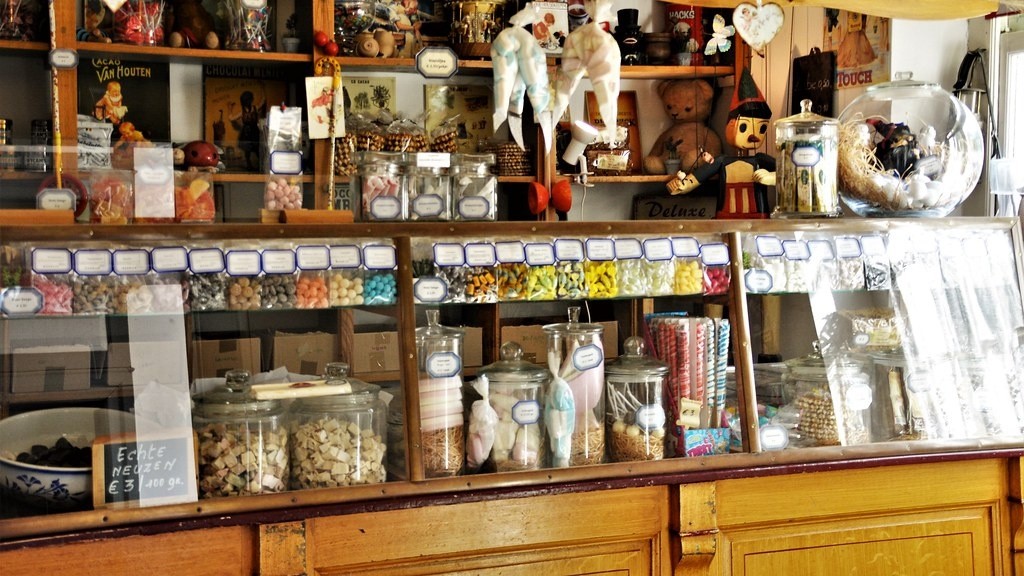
<box><xmin>642</xmin><ymin>79</ymin><xmax>723</xmax><ymax>175</ymax></box>
<box><xmin>165</xmin><ymin>0</ymin><xmax>221</xmax><ymax>49</ymax></box>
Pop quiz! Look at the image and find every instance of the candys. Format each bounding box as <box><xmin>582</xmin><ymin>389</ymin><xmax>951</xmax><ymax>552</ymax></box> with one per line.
<box><xmin>0</xmin><ymin>257</ymin><xmax>734</xmax><ymax>313</ymax></box>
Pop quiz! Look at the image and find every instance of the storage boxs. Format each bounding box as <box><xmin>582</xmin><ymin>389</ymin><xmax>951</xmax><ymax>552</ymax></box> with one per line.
<box><xmin>255</xmin><ymin>327</ymin><xmax>339</xmax><ymax>375</ymax></box>
<box><xmin>96</xmin><ymin>337</ymin><xmax>177</xmax><ymax>386</ymax></box>
<box><xmin>448</xmin><ymin>325</ymin><xmax>483</xmax><ymax>368</ymax></box>
<box><xmin>10</xmin><ymin>336</ymin><xmax>97</xmax><ymax>394</ymax></box>
<box><xmin>353</xmin><ymin>332</ymin><xmax>399</xmax><ymax>374</ymax></box>
<box><xmin>500</xmin><ymin>314</ymin><xmax>619</xmax><ymax>362</ymax></box>
<box><xmin>632</xmin><ymin>193</ymin><xmax>718</xmax><ymax>217</ymax></box>
<box><xmin>192</xmin><ymin>330</ymin><xmax>260</xmax><ymax>381</ymax></box>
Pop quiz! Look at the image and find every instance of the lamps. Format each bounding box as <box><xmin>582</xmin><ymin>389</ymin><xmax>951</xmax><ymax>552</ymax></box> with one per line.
<box><xmin>561</xmin><ymin>120</ymin><xmax>599</xmax><ymax>187</ymax></box>
<box><xmin>951</xmin><ymin>47</ymin><xmax>988</xmax><ymax>131</ymax></box>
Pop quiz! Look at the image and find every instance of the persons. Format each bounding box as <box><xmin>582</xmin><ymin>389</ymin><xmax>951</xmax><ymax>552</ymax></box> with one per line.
<box><xmin>95</xmin><ymin>81</ymin><xmax>129</xmax><ymax>125</ymax></box>
<box><xmin>114</xmin><ymin>121</ymin><xmax>153</xmax><ymax>156</ymax></box>
<box><xmin>665</xmin><ymin>64</ymin><xmax>776</xmax><ymax>219</ymax></box>
<box><xmin>229</xmin><ymin>91</ymin><xmax>267</xmax><ymax>172</ymax></box>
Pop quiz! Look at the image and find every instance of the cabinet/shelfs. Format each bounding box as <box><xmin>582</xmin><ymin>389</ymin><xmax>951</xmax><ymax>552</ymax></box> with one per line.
<box><xmin>0</xmin><ymin>216</ymin><xmax>1024</xmax><ymax>576</ymax></box>
<box><xmin>0</xmin><ymin>0</ymin><xmax>753</xmax><ymax>418</ymax></box>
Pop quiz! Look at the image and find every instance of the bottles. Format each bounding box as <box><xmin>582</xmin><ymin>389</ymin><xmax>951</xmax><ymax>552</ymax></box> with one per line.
<box><xmin>836</xmin><ymin>69</ymin><xmax>984</xmax><ymax>219</ymax></box>
<box><xmin>0</xmin><ymin>118</ymin><xmax>24</xmax><ymax>171</ymax></box>
<box><xmin>23</xmin><ymin>119</ymin><xmax>54</xmax><ymax>172</ymax></box>
<box><xmin>356</xmin><ymin>150</ymin><xmax>499</xmax><ymax>222</ymax></box>
<box><xmin>864</xmin><ymin>325</ymin><xmax>920</xmax><ymax>442</ymax></box>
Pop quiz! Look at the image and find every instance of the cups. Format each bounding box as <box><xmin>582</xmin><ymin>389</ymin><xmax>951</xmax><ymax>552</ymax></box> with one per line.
<box><xmin>191</xmin><ymin>367</ymin><xmax>290</xmax><ymax>500</ymax></box>
<box><xmin>473</xmin><ymin>340</ymin><xmax>554</xmax><ymax>473</ymax></box>
<box><xmin>603</xmin><ymin>334</ymin><xmax>670</xmax><ymax>464</ymax></box>
<box><xmin>411</xmin><ymin>308</ymin><xmax>467</xmax><ymax>478</ymax></box>
<box><xmin>289</xmin><ymin>362</ymin><xmax>389</xmax><ymax>488</ymax></box>
<box><xmin>773</xmin><ymin>99</ymin><xmax>841</xmax><ymax>220</ymax></box>
<box><xmin>783</xmin><ymin>339</ymin><xmax>874</xmax><ymax>450</ymax></box>
<box><xmin>541</xmin><ymin>306</ymin><xmax>606</xmax><ymax>467</ymax></box>
<box><xmin>25</xmin><ymin>230</ymin><xmax>892</xmax><ymax>318</ymax></box>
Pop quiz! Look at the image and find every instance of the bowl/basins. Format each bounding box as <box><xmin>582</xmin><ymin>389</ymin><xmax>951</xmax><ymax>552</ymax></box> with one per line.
<box><xmin>1</xmin><ymin>406</ymin><xmax>168</xmax><ymax>508</ymax></box>
